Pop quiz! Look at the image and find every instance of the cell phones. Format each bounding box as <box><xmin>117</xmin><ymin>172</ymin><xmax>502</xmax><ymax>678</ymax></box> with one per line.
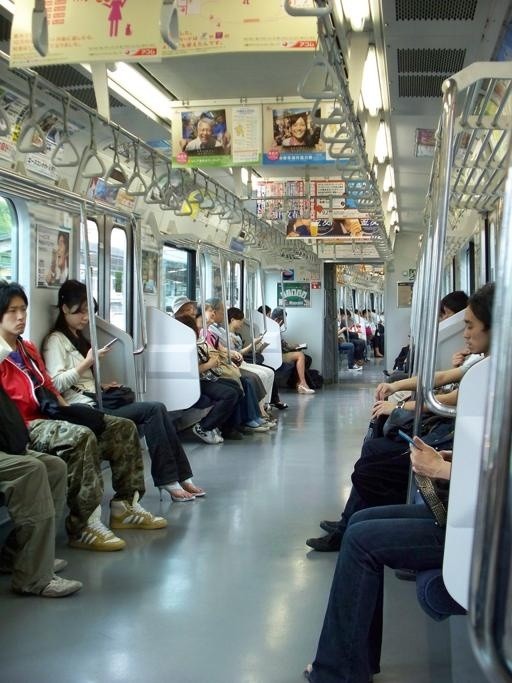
<box><xmin>102</xmin><ymin>337</ymin><xmax>118</xmax><ymax>350</ymax></box>
<box><xmin>258</xmin><ymin>329</ymin><xmax>267</xmax><ymax>339</ymax></box>
<box><xmin>398</xmin><ymin>428</ymin><xmax>421</xmax><ymax>449</ymax></box>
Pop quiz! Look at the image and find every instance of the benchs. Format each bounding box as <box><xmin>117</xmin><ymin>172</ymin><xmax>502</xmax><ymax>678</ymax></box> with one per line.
<box><xmin>406</xmin><ymin>316</ymin><xmax>512</xmax><ymax>618</ymax></box>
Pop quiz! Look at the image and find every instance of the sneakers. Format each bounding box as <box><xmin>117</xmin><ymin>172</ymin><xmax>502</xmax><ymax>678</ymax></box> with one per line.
<box><xmin>222</xmin><ymin>426</ymin><xmax>252</xmax><ymax>440</ymax></box>
<box><xmin>306</xmin><ymin>518</ymin><xmax>345</xmax><ymax>551</ymax></box>
<box><xmin>67</xmin><ymin>503</ymin><xmax>126</xmax><ymax>551</ymax></box>
<box><xmin>40</xmin><ymin>556</ymin><xmax>83</xmax><ymax>597</ymax></box>
<box><xmin>193</xmin><ymin>422</ymin><xmax>225</xmax><ymax>445</ymax></box>
<box><xmin>109</xmin><ymin>489</ymin><xmax>168</xmax><ymax>529</ymax></box>
<box><xmin>348</xmin><ymin>363</ymin><xmax>364</xmax><ymax>370</ymax></box>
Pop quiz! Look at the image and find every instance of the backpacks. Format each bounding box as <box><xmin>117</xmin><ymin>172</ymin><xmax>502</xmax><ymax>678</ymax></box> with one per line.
<box><xmin>195</xmin><ymin>337</ymin><xmax>223</xmax><ymax>382</ymax></box>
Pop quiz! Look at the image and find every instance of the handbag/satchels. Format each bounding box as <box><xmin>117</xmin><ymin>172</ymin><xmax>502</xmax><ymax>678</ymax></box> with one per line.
<box><xmin>244</xmin><ymin>350</ymin><xmax>264</xmax><ymax>365</ymax></box>
<box><xmin>413</xmin><ymin>451</ymin><xmax>454</xmax><ymax>528</ymax></box>
<box><xmin>97</xmin><ymin>385</ymin><xmax>135</xmax><ymax>409</ymax></box>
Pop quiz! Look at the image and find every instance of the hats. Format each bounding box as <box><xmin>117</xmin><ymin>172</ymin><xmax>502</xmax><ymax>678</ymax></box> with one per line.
<box><xmin>169</xmin><ymin>294</ymin><xmax>198</xmax><ymax>317</ymax></box>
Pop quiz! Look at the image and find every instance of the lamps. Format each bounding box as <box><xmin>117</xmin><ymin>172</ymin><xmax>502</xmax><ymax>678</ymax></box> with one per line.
<box><xmin>334</xmin><ymin>0</ymin><xmax>400</xmax><ymax>246</ymax></box>
<box><xmin>108</xmin><ymin>60</ymin><xmax>176</xmax><ymax>127</ymax></box>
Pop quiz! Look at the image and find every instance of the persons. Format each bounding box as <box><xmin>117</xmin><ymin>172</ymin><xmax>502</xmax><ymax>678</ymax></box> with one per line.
<box><xmin>306</xmin><ymin>283</ymin><xmax>497</xmax><ymax>682</ymax></box>
<box><xmin>273</xmin><ymin>108</ymin><xmax>321</xmax><ymax>147</ymax></box>
<box><xmin>42</xmin><ymin>279</ymin><xmax>206</xmax><ymax>502</ymax></box>
<box><xmin>306</xmin><ymin>289</ymin><xmax>490</xmax><ymax>552</ymax></box>
<box><xmin>0</xmin><ymin>278</ymin><xmax>167</xmax><ymax>551</ymax></box>
<box><xmin>172</xmin><ymin>296</ymin><xmax>315</xmax><ymax>444</ymax></box>
<box><xmin>0</xmin><ymin>383</ymin><xmax>83</xmax><ymax>598</ymax></box>
<box><xmin>182</xmin><ymin>112</ymin><xmax>227</xmax><ymax>151</ymax></box>
<box><xmin>45</xmin><ymin>232</ymin><xmax>69</xmax><ymax>286</ymax></box>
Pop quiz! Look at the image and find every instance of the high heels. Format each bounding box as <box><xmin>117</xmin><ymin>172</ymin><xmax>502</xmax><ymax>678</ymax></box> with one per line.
<box><xmin>157</xmin><ymin>478</ymin><xmax>206</xmax><ymax>502</ymax></box>
<box><xmin>245</xmin><ymin>401</ymin><xmax>288</xmax><ymax>432</ymax></box>
<box><xmin>296</xmin><ymin>382</ymin><xmax>316</xmax><ymax>394</ymax></box>
